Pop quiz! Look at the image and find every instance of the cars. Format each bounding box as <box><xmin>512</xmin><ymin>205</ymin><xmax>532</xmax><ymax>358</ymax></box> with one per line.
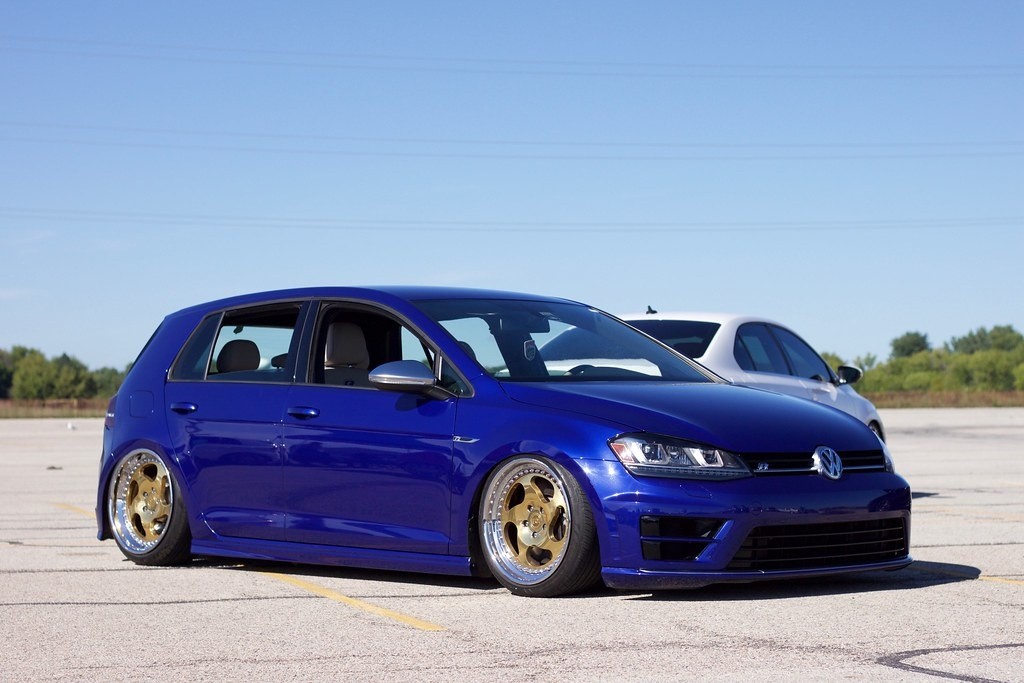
<box><xmin>494</xmin><ymin>305</ymin><xmax>888</xmax><ymax>447</ymax></box>
<box><xmin>95</xmin><ymin>286</ymin><xmax>910</xmax><ymax>591</ymax></box>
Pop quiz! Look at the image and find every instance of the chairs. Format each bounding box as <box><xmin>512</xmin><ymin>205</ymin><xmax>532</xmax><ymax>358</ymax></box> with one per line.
<box><xmin>354</xmin><ymin>348</ymin><xmax>372</xmax><ymax>389</ymax></box>
<box><xmin>270</xmin><ymin>352</ymin><xmax>290</xmax><ymax>384</ymax></box>
<box><xmin>434</xmin><ymin>341</ymin><xmax>476</xmax><ymax>397</ymax></box>
<box><xmin>673</xmin><ymin>343</ymin><xmax>706</xmax><ymax>359</ymax></box>
<box><xmin>323</xmin><ymin>321</ymin><xmax>374</xmax><ymax>388</ymax></box>
<box><xmin>215</xmin><ymin>340</ymin><xmax>261</xmax><ymax>382</ymax></box>
